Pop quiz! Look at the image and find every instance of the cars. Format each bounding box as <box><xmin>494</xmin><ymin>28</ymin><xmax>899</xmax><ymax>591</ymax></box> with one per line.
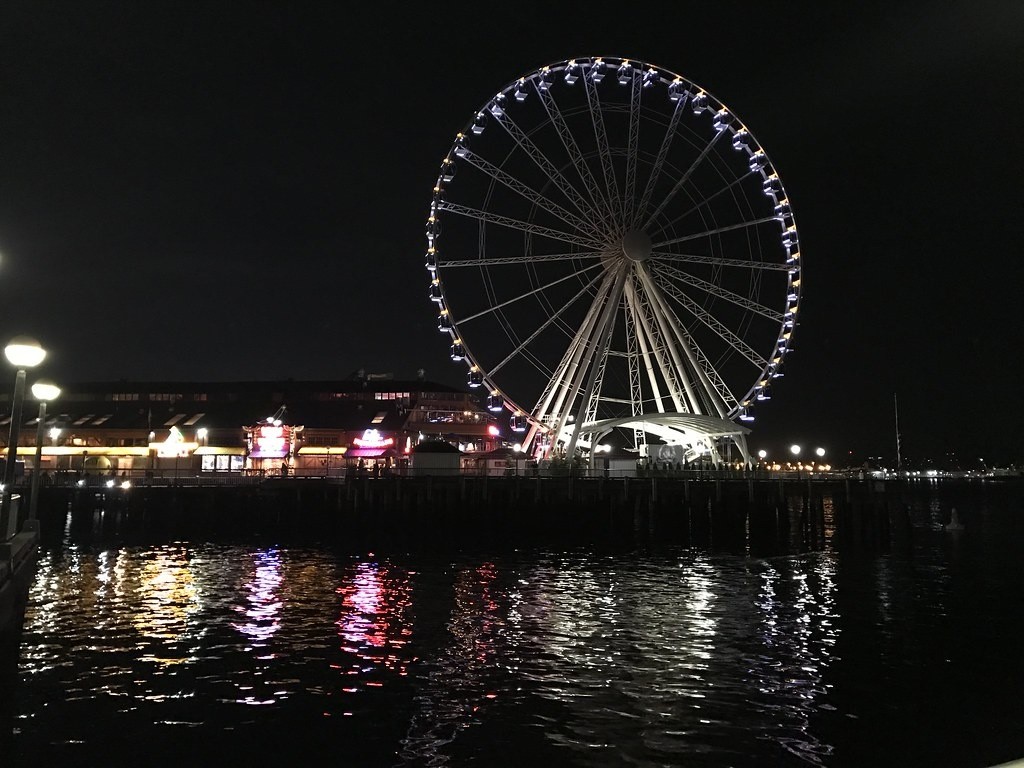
<box><xmin>842</xmin><ymin>473</ymin><xmax>859</xmax><ymax>480</ymax></box>
<box><xmin>819</xmin><ymin>473</ymin><xmax>836</xmax><ymax>481</ymax></box>
<box><xmin>880</xmin><ymin>471</ymin><xmax>897</xmax><ymax>478</ymax></box>
<box><xmin>784</xmin><ymin>474</ymin><xmax>801</xmax><ymax>478</ymax></box>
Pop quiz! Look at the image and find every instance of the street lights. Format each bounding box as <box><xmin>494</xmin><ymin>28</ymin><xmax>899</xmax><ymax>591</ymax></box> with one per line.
<box><xmin>0</xmin><ymin>336</ymin><xmax>50</xmax><ymax>541</ymax></box>
<box><xmin>894</xmin><ymin>393</ymin><xmax>901</xmax><ymax>468</ymax></box>
<box><xmin>326</xmin><ymin>445</ymin><xmax>330</xmax><ymax>475</ymax></box>
<box><xmin>345</xmin><ymin>442</ymin><xmax>351</xmax><ymax>477</ymax></box>
<box><xmin>604</xmin><ymin>445</ymin><xmax>611</xmax><ymax>477</ymax></box>
<box><xmin>28</xmin><ymin>378</ymin><xmax>63</xmax><ymax>520</ymax></box>
